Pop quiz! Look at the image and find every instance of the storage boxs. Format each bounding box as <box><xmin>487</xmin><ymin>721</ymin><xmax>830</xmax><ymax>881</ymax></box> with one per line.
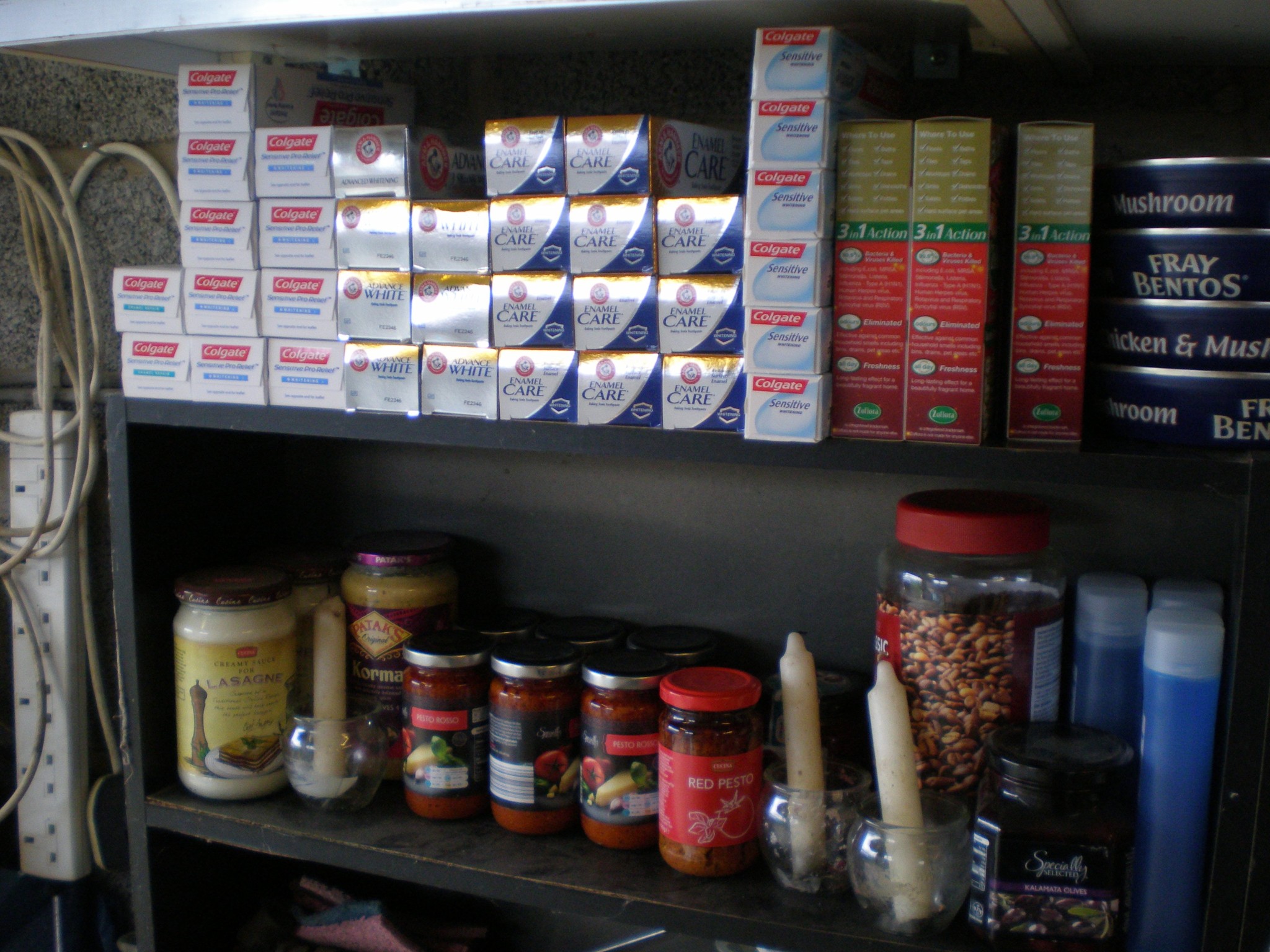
<box><xmin>114</xmin><ymin>34</ymin><xmax>1093</xmax><ymax>441</ymax></box>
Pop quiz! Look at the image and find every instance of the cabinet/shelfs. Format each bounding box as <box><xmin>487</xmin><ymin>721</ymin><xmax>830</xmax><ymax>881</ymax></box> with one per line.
<box><xmin>97</xmin><ymin>389</ymin><xmax>1269</xmax><ymax>952</ymax></box>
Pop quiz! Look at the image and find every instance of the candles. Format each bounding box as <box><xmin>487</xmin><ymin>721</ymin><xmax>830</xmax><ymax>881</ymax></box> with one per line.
<box><xmin>312</xmin><ymin>587</ymin><xmax>346</xmax><ymax>776</ymax></box>
<box><xmin>779</xmin><ymin>630</ymin><xmax>826</xmax><ymax>872</ymax></box>
<box><xmin>868</xmin><ymin>660</ymin><xmax>937</xmax><ymax>924</ymax></box>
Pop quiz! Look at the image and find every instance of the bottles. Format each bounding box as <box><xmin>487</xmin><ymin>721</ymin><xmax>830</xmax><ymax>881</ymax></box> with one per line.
<box><xmin>282</xmin><ymin>696</ymin><xmax>393</xmax><ymax>815</ymax></box>
<box><xmin>757</xmin><ymin>760</ymin><xmax>870</xmax><ymax>895</ymax></box>
<box><xmin>846</xmin><ymin>793</ymin><xmax>974</xmax><ymax>938</ymax></box>
<box><xmin>1069</xmin><ymin>572</ymin><xmax>1226</xmax><ymax>952</ymax></box>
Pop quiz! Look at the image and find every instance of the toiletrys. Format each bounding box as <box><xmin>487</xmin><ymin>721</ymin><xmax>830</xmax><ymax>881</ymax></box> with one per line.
<box><xmin>105</xmin><ymin>17</ymin><xmax>840</xmax><ymax>449</ymax></box>
